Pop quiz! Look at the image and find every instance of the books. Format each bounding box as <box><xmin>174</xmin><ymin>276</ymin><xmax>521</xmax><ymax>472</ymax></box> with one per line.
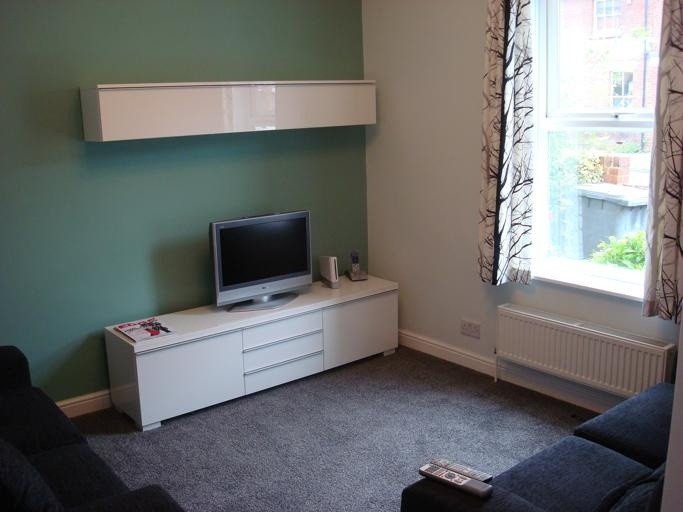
<box><xmin>114</xmin><ymin>317</ymin><xmax>176</xmax><ymax>344</ymax></box>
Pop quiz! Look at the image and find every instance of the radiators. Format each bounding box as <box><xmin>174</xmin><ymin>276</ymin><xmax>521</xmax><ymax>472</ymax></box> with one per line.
<box><xmin>492</xmin><ymin>299</ymin><xmax>675</xmax><ymax>399</ymax></box>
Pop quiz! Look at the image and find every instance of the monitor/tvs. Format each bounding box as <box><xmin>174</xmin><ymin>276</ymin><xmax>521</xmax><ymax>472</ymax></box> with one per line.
<box><xmin>209</xmin><ymin>208</ymin><xmax>313</xmax><ymax>313</ymax></box>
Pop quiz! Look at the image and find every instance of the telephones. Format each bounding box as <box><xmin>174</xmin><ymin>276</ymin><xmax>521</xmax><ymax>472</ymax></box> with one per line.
<box><xmin>345</xmin><ymin>250</ymin><xmax>368</xmax><ymax>281</ymax></box>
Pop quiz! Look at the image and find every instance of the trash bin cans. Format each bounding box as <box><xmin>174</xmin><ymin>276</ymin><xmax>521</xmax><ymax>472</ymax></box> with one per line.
<box><xmin>577</xmin><ymin>184</ymin><xmax>650</xmax><ymax>258</ymax></box>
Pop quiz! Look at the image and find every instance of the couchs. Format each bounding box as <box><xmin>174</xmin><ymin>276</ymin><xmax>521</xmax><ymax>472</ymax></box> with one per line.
<box><xmin>396</xmin><ymin>381</ymin><xmax>674</xmax><ymax>510</ymax></box>
<box><xmin>1</xmin><ymin>338</ymin><xmax>183</xmax><ymax>510</ymax></box>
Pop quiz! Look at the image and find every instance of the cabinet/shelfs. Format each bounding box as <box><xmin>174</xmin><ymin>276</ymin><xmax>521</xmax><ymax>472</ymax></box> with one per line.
<box><xmin>100</xmin><ymin>270</ymin><xmax>400</xmax><ymax>435</ymax></box>
<box><xmin>80</xmin><ymin>77</ymin><xmax>377</xmax><ymax>147</ymax></box>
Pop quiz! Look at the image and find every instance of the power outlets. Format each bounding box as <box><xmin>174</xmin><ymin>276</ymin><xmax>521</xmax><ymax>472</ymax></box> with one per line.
<box><xmin>459</xmin><ymin>319</ymin><xmax>481</xmax><ymax>338</ymax></box>
